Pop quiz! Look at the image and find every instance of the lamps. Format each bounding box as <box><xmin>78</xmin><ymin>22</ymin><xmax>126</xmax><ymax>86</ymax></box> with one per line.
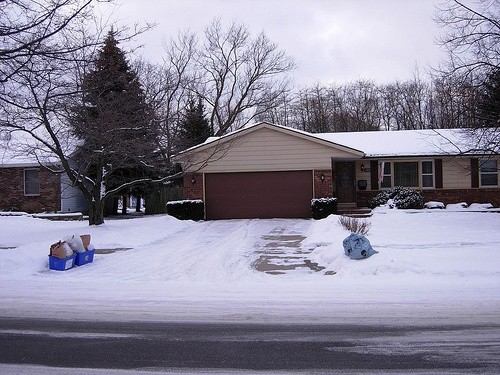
<box><xmin>320</xmin><ymin>173</ymin><xmax>325</xmax><ymax>182</ymax></box>
<box><xmin>361</xmin><ymin>163</ymin><xmax>364</xmax><ymax>172</ymax></box>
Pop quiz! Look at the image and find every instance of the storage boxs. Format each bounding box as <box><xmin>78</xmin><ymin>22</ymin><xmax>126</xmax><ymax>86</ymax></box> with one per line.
<box><xmin>48</xmin><ymin>252</ymin><xmax>77</xmax><ymax>271</ymax></box>
<box><xmin>73</xmin><ymin>248</ymin><xmax>95</xmax><ymax>267</ymax></box>
